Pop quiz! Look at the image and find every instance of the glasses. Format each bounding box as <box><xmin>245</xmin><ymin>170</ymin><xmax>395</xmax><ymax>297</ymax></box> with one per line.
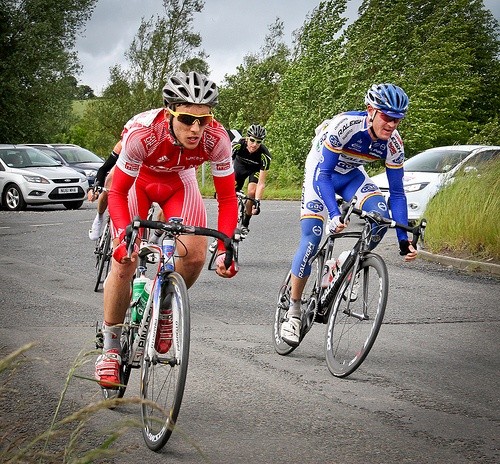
<box><xmin>377</xmin><ymin>109</ymin><xmax>400</xmax><ymax>124</ymax></box>
<box><xmin>166</xmin><ymin>106</ymin><xmax>214</xmax><ymax>125</ymax></box>
<box><xmin>248</xmin><ymin>137</ymin><xmax>262</xmax><ymax>144</ymax></box>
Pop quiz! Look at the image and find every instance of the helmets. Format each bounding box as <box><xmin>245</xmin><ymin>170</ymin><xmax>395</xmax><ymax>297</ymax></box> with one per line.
<box><xmin>364</xmin><ymin>83</ymin><xmax>409</xmax><ymax>119</ymax></box>
<box><xmin>246</xmin><ymin>123</ymin><xmax>267</xmax><ymax>140</ymax></box>
<box><xmin>162</xmin><ymin>70</ymin><xmax>220</xmax><ymax>107</ymax></box>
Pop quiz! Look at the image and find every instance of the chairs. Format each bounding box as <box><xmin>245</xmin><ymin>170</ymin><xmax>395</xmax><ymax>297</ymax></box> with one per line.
<box><xmin>6</xmin><ymin>154</ymin><xmax>21</xmax><ymax>164</ymax></box>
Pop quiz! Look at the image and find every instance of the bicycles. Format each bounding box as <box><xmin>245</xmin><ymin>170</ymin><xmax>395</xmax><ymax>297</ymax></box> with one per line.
<box><xmin>94</xmin><ymin>215</ymin><xmax>242</xmax><ymax>452</ymax></box>
<box><xmin>89</xmin><ymin>176</ymin><xmax>115</xmax><ymax>292</ymax></box>
<box><xmin>206</xmin><ymin>188</ymin><xmax>261</xmax><ymax>272</ymax></box>
<box><xmin>271</xmin><ymin>195</ymin><xmax>429</xmax><ymax>379</ymax></box>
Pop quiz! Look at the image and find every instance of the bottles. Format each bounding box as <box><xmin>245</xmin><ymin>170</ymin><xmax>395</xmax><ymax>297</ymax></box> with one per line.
<box><xmin>320</xmin><ymin>250</ymin><xmax>351</xmax><ymax>288</ymax></box>
<box><xmin>130</xmin><ymin>274</ymin><xmax>154</xmax><ymax>323</ymax></box>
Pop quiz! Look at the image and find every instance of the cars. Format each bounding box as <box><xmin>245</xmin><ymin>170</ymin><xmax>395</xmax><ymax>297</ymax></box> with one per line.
<box><xmin>24</xmin><ymin>141</ymin><xmax>106</xmax><ymax>193</ymax></box>
<box><xmin>370</xmin><ymin>144</ymin><xmax>500</xmax><ymax>231</ymax></box>
<box><xmin>0</xmin><ymin>143</ymin><xmax>89</xmax><ymax>211</ymax></box>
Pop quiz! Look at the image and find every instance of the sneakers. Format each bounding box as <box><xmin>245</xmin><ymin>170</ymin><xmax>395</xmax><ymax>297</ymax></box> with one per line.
<box><xmin>154</xmin><ymin>310</ymin><xmax>174</xmax><ymax>353</ymax></box>
<box><xmin>240</xmin><ymin>225</ymin><xmax>250</xmax><ymax>236</ymax></box>
<box><xmin>88</xmin><ymin>212</ymin><xmax>108</xmax><ymax>240</ymax></box>
<box><xmin>343</xmin><ymin>271</ymin><xmax>361</xmax><ymax>303</ymax></box>
<box><xmin>209</xmin><ymin>238</ymin><xmax>219</xmax><ymax>253</ymax></box>
<box><xmin>94</xmin><ymin>348</ymin><xmax>123</xmax><ymax>389</ymax></box>
<box><xmin>280</xmin><ymin>311</ymin><xmax>302</xmax><ymax>346</ymax></box>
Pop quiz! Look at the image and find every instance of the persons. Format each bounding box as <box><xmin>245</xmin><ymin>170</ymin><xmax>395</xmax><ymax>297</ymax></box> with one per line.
<box><xmin>208</xmin><ymin>124</ymin><xmax>272</xmax><ymax>254</ymax></box>
<box><xmin>88</xmin><ymin>140</ymin><xmax>123</xmax><ymax>241</ymax></box>
<box><xmin>94</xmin><ymin>70</ymin><xmax>238</xmax><ymax>389</ymax></box>
<box><xmin>282</xmin><ymin>84</ymin><xmax>417</xmax><ymax>348</ymax></box>
